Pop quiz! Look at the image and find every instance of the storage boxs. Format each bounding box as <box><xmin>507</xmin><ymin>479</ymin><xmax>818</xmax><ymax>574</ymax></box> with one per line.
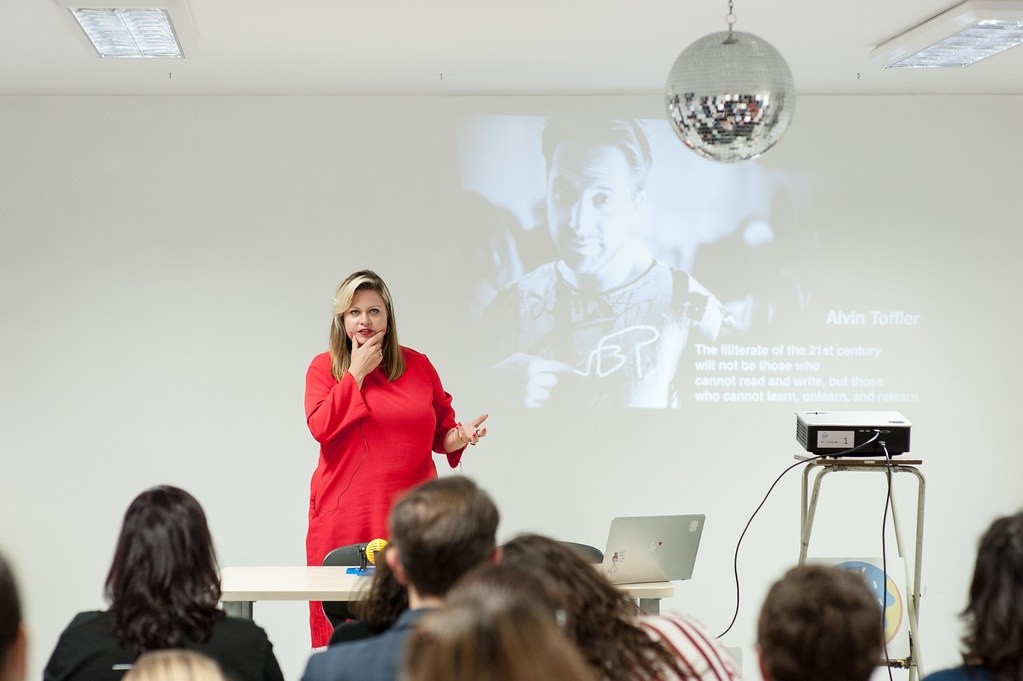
<box><xmin>806</xmin><ymin>556</ymin><xmax>911</xmax><ymax>659</ymax></box>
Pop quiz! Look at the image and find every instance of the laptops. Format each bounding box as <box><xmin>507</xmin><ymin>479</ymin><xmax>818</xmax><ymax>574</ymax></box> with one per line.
<box><xmin>590</xmin><ymin>513</ymin><xmax>706</xmax><ymax>585</ymax></box>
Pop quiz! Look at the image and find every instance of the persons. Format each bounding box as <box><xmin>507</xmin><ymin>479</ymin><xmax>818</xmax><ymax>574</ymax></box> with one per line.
<box><xmin>298</xmin><ymin>470</ymin><xmax>749</xmax><ymax>681</ymax></box>
<box><xmin>121</xmin><ymin>648</ymin><xmax>225</xmax><ymax>681</ymax></box>
<box><xmin>43</xmin><ymin>484</ymin><xmax>284</xmax><ymax>681</ymax></box>
<box><xmin>919</xmin><ymin>508</ymin><xmax>1023</xmax><ymax>681</ymax></box>
<box><xmin>754</xmin><ymin>563</ymin><xmax>883</xmax><ymax>681</ymax></box>
<box><xmin>0</xmin><ymin>551</ymin><xmax>28</xmax><ymax>681</ymax></box>
<box><xmin>478</xmin><ymin>107</ymin><xmax>747</xmax><ymax>412</ymax></box>
<box><xmin>305</xmin><ymin>271</ymin><xmax>491</xmax><ymax>649</ymax></box>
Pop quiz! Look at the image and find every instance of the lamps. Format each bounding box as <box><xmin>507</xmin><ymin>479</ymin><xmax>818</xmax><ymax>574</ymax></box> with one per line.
<box><xmin>53</xmin><ymin>0</ymin><xmax>202</xmax><ymax>69</ymax></box>
<box><xmin>869</xmin><ymin>0</ymin><xmax>1023</xmax><ymax>69</ymax></box>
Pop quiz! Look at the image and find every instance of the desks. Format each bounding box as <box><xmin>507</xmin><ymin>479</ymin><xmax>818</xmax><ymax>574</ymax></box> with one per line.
<box><xmin>793</xmin><ymin>454</ymin><xmax>926</xmax><ymax>681</ymax></box>
<box><xmin>217</xmin><ymin>566</ymin><xmax>674</xmax><ymax>621</ymax></box>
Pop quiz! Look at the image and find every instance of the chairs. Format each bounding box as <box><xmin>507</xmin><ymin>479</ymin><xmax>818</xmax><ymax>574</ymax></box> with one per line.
<box><xmin>321</xmin><ymin>543</ymin><xmax>370</xmax><ymax>629</ymax></box>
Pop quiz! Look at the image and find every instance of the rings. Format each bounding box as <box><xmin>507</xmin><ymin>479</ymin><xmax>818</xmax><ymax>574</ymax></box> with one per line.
<box><xmin>378</xmin><ymin>350</ymin><xmax>382</xmax><ymax>356</ymax></box>
<box><xmin>476</xmin><ymin>429</ymin><xmax>481</xmax><ymax>438</ymax></box>
<box><xmin>470</xmin><ymin>441</ymin><xmax>476</xmax><ymax>446</ymax></box>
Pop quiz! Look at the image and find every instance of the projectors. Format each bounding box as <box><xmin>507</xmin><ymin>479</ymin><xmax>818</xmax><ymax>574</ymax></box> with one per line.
<box><xmin>794</xmin><ymin>410</ymin><xmax>912</xmax><ymax>460</ymax></box>
